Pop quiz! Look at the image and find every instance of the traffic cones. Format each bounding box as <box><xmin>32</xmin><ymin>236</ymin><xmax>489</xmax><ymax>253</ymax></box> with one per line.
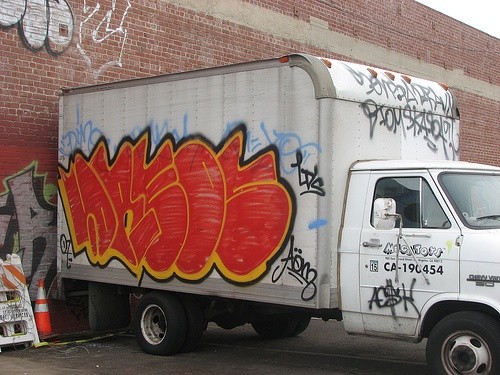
<box><xmin>34</xmin><ymin>280</ymin><xmax>59</xmax><ymax>344</ymax></box>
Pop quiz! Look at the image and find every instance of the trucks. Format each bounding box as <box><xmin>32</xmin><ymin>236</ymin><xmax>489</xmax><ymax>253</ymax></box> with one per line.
<box><xmin>56</xmin><ymin>53</ymin><xmax>500</xmax><ymax>375</ymax></box>
